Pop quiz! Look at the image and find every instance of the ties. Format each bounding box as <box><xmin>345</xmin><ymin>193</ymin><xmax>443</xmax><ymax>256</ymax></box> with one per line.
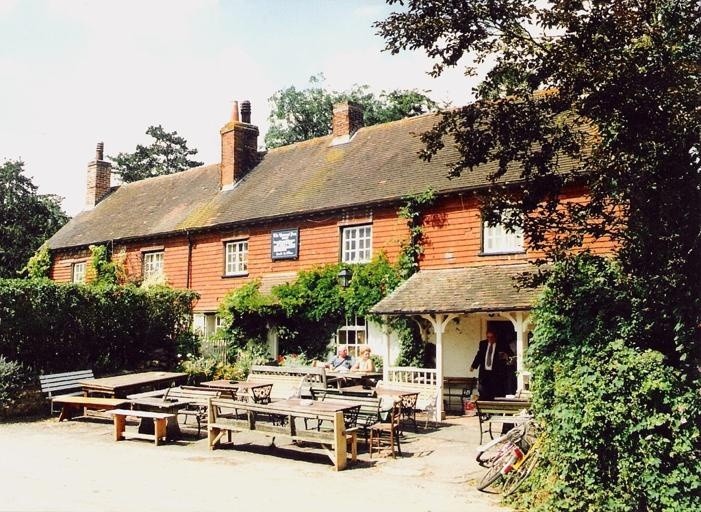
<box><xmin>486</xmin><ymin>343</ymin><xmax>494</xmax><ymax>367</ymax></box>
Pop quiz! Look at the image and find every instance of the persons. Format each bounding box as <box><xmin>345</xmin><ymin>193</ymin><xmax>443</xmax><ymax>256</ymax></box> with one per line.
<box><xmin>328</xmin><ymin>346</ymin><xmax>352</xmax><ymax>369</ymax></box>
<box><xmin>349</xmin><ymin>346</ymin><xmax>374</xmax><ymax>372</ymax></box>
<box><xmin>470</xmin><ymin>329</ymin><xmax>513</xmax><ymax>421</ymax></box>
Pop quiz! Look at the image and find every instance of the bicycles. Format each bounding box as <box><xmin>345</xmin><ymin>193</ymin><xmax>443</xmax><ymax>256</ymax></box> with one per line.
<box><xmin>477</xmin><ymin>420</ymin><xmax>543</xmax><ymax>498</ymax></box>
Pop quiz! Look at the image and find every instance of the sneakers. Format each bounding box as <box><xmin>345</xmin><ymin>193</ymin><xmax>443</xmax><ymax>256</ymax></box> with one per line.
<box><xmin>481</xmin><ymin>412</ymin><xmax>491</xmax><ymax>421</ymax></box>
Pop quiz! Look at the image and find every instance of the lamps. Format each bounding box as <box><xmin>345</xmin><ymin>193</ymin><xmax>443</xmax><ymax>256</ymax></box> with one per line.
<box><xmin>337</xmin><ymin>267</ymin><xmax>352</xmax><ymax>287</ymax></box>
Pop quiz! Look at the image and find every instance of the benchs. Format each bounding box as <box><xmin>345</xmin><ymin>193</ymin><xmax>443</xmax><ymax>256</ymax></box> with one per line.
<box><xmin>426</xmin><ymin>377</ymin><xmax>477</xmax><ymax>409</ymax></box>
<box><xmin>39</xmin><ymin>368</ymin><xmax>442</xmax><ymax>472</ymax></box>
<box><xmin>475</xmin><ymin>399</ymin><xmax>533</xmax><ymax>445</ymax></box>
